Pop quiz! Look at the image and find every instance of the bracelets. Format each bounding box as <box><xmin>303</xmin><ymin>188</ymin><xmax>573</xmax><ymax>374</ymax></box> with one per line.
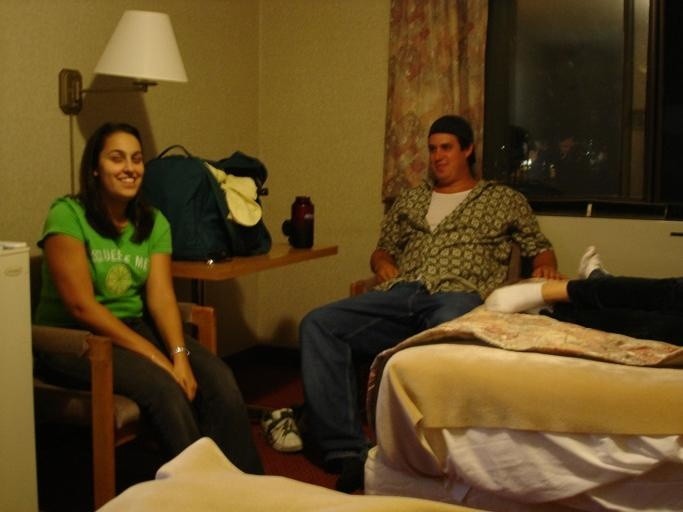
<box><xmin>169</xmin><ymin>345</ymin><xmax>190</xmax><ymax>357</ymax></box>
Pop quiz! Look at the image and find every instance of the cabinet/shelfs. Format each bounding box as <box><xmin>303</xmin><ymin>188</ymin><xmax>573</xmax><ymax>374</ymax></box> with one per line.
<box><xmin>1</xmin><ymin>241</ymin><xmax>42</xmax><ymax>512</ymax></box>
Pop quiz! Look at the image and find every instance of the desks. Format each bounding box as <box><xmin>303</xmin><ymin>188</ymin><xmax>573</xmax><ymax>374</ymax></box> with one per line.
<box><xmin>171</xmin><ymin>242</ymin><xmax>338</xmax><ymax>421</ymax></box>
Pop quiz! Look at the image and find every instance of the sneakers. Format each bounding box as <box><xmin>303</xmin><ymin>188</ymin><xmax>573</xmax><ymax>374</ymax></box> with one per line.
<box><xmin>260</xmin><ymin>406</ymin><xmax>304</xmax><ymax>455</ymax></box>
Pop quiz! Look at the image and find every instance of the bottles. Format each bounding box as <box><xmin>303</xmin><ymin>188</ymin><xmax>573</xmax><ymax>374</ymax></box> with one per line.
<box><xmin>290</xmin><ymin>196</ymin><xmax>315</xmax><ymax>249</ymax></box>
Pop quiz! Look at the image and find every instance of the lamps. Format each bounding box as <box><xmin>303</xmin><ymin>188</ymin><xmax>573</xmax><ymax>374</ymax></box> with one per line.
<box><xmin>60</xmin><ymin>10</ymin><xmax>190</xmax><ymax>112</ymax></box>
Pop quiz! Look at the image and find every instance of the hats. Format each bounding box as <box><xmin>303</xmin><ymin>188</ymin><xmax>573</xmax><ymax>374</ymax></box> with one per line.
<box><xmin>427</xmin><ymin>113</ymin><xmax>476</xmax><ymax>164</ymax></box>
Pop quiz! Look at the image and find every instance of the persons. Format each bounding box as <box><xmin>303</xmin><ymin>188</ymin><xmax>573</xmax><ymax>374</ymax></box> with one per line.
<box><xmin>299</xmin><ymin>116</ymin><xmax>564</xmax><ymax>494</ymax></box>
<box><xmin>35</xmin><ymin>121</ymin><xmax>260</xmax><ymax>479</ymax></box>
<box><xmin>482</xmin><ymin>260</ymin><xmax>680</xmax><ymax>351</ymax></box>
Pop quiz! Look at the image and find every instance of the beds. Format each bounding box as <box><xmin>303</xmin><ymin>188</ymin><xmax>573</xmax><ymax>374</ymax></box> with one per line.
<box><xmin>85</xmin><ymin>435</ymin><xmax>484</xmax><ymax>511</ymax></box>
<box><xmin>366</xmin><ymin>297</ymin><xmax>682</xmax><ymax>512</ymax></box>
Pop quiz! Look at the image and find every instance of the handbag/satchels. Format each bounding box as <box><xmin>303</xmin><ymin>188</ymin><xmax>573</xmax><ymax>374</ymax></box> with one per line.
<box><xmin>142</xmin><ymin>144</ymin><xmax>273</xmax><ymax>261</ymax></box>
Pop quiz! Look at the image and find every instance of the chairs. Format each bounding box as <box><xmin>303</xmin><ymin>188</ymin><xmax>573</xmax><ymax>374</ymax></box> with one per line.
<box><xmin>29</xmin><ymin>258</ymin><xmax>219</xmax><ymax>511</ymax></box>
